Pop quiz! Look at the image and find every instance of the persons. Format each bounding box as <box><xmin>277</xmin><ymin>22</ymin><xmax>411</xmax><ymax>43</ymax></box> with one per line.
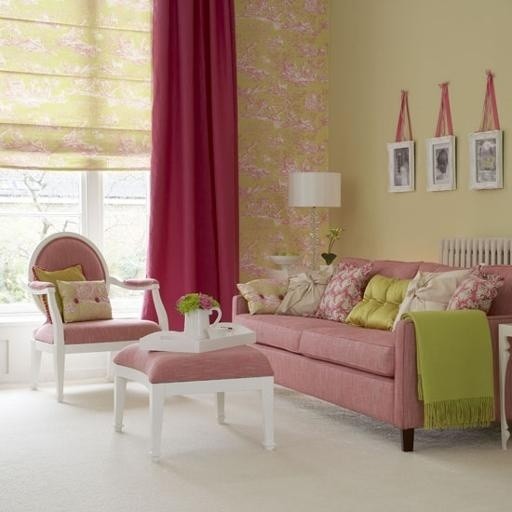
<box><xmin>435</xmin><ymin>149</ymin><xmax>449</xmax><ymax>180</ymax></box>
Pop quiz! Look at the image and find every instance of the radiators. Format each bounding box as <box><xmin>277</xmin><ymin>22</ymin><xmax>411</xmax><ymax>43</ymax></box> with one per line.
<box><xmin>439</xmin><ymin>238</ymin><xmax>512</xmax><ymax>268</ymax></box>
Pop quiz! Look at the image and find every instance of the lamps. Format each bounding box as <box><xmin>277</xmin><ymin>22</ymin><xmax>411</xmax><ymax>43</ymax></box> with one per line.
<box><xmin>289</xmin><ymin>170</ymin><xmax>342</xmax><ymax>281</ymax></box>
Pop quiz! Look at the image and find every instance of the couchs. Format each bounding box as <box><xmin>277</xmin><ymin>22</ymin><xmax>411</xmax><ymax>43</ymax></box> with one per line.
<box><xmin>231</xmin><ymin>255</ymin><xmax>512</xmax><ymax>454</ymax></box>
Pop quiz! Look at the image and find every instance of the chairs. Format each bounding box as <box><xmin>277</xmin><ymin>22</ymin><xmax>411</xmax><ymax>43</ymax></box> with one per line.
<box><xmin>26</xmin><ymin>231</ymin><xmax>170</xmax><ymax>403</ymax></box>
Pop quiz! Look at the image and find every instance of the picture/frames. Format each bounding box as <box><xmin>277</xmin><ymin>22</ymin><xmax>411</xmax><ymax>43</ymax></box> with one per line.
<box><xmin>471</xmin><ymin>128</ymin><xmax>502</xmax><ymax>191</ymax></box>
<box><xmin>387</xmin><ymin>141</ymin><xmax>416</xmax><ymax>194</ymax></box>
<box><xmin>427</xmin><ymin>134</ymin><xmax>455</xmax><ymax>191</ymax></box>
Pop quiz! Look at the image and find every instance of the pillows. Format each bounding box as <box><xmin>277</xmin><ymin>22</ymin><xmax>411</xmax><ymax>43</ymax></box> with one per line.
<box><xmin>236</xmin><ymin>278</ymin><xmax>289</xmax><ymax>316</ymax></box>
<box><xmin>55</xmin><ymin>279</ymin><xmax>113</xmax><ymax>322</ymax></box>
<box><xmin>392</xmin><ymin>270</ymin><xmax>471</xmax><ymax>331</ymax></box>
<box><xmin>344</xmin><ymin>272</ymin><xmax>413</xmax><ymax>331</ymax></box>
<box><xmin>315</xmin><ymin>261</ymin><xmax>375</xmax><ymax>323</ymax></box>
<box><xmin>276</xmin><ymin>273</ymin><xmax>331</xmax><ymax>315</ymax></box>
<box><xmin>445</xmin><ymin>272</ymin><xmax>505</xmax><ymax>314</ymax></box>
<box><xmin>31</xmin><ymin>265</ymin><xmax>87</xmax><ymax>319</ymax></box>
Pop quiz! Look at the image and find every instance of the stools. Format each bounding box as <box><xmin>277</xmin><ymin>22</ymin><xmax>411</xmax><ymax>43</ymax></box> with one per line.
<box><xmin>111</xmin><ymin>343</ymin><xmax>276</xmax><ymax>469</ymax></box>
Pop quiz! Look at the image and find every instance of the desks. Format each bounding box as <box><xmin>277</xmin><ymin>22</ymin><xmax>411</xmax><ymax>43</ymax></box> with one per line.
<box><xmin>497</xmin><ymin>322</ymin><xmax>512</xmax><ymax>451</ymax></box>
